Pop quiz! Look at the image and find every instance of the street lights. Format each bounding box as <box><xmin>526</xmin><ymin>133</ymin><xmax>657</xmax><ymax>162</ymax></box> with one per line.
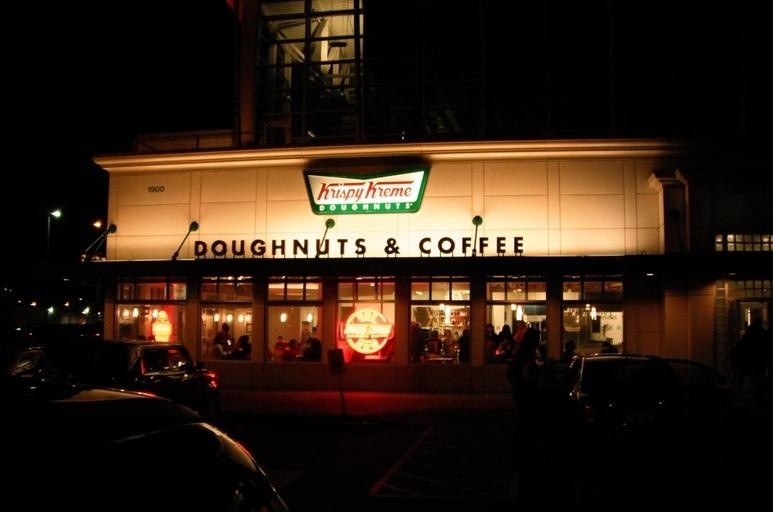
<box><xmin>41</xmin><ymin>209</ymin><xmax>61</xmax><ymax>324</ymax></box>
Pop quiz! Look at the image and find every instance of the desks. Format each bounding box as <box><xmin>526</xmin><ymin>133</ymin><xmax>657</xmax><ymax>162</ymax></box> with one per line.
<box><xmin>41</xmin><ymin>209</ymin><xmax>61</xmax><ymax>324</ymax></box>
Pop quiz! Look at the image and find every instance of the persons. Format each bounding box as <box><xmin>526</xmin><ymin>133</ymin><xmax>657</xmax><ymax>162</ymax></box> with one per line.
<box><xmin>411</xmin><ymin>320</ymin><xmax>619</xmax><ymax>365</ymax></box>
<box><xmin>206</xmin><ymin>322</ymin><xmax>321</xmax><ymax>362</ymax></box>
<box><xmin>508</xmin><ymin>327</ymin><xmax>546</xmax><ymax>451</ymax></box>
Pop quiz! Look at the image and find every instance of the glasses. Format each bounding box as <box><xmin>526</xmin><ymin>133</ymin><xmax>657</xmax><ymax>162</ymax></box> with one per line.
<box><xmin>471</xmin><ymin>215</ymin><xmax>482</xmax><ymax>257</ymax></box>
<box><xmin>314</xmin><ymin>218</ymin><xmax>335</xmax><ymax>258</ymax></box>
<box><xmin>172</xmin><ymin>221</ymin><xmax>198</xmax><ymax>261</ymax></box>
<box><xmin>86</xmin><ymin>224</ymin><xmax>117</xmax><ymax>258</ymax></box>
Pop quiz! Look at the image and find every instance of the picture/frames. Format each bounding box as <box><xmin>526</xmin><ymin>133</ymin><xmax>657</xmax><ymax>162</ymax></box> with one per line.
<box><xmin>427</xmin><ymin>358</ymin><xmax>453</xmax><ymax>364</ymax></box>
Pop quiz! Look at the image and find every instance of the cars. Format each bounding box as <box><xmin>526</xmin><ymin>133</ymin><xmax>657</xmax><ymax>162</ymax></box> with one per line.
<box><xmin>128</xmin><ymin>342</ymin><xmax>220</xmax><ymax>410</ymax></box>
<box><xmin>539</xmin><ymin>352</ymin><xmax>754</xmax><ymax>455</ymax></box>
<box><xmin>0</xmin><ymin>344</ymin><xmax>289</xmax><ymax>511</ymax></box>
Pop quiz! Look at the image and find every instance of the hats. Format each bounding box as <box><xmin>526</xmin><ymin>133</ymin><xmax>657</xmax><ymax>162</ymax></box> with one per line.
<box><xmin>539</xmin><ymin>352</ymin><xmax>754</xmax><ymax>455</ymax></box>
